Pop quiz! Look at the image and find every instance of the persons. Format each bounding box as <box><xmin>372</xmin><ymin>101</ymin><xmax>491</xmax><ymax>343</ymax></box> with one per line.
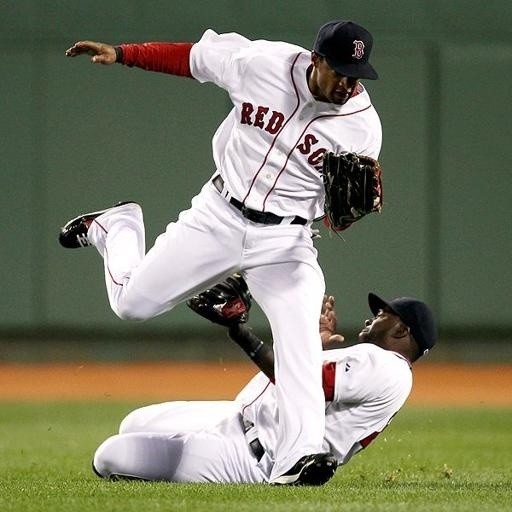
<box><xmin>57</xmin><ymin>19</ymin><xmax>382</xmax><ymax>487</ymax></box>
<box><xmin>93</xmin><ymin>272</ymin><xmax>437</xmax><ymax>485</ymax></box>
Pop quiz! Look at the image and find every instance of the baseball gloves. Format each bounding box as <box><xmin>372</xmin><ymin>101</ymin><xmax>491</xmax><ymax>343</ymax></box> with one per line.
<box><xmin>322</xmin><ymin>152</ymin><xmax>382</xmax><ymax>231</ymax></box>
<box><xmin>188</xmin><ymin>272</ymin><xmax>254</xmax><ymax>325</ymax></box>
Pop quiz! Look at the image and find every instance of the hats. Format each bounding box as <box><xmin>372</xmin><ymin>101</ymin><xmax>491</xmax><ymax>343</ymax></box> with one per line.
<box><xmin>367</xmin><ymin>292</ymin><xmax>436</xmax><ymax>356</ymax></box>
<box><xmin>313</xmin><ymin>20</ymin><xmax>378</xmax><ymax>81</ymax></box>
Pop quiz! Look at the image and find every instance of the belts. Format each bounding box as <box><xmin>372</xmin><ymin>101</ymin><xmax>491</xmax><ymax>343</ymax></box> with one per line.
<box><xmin>212</xmin><ymin>176</ymin><xmax>306</xmax><ymax>225</ymax></box>
<box><xmin>243</xmin><ymin>421</ymin><xmax>264</xmax><ymax>461</ymax></box>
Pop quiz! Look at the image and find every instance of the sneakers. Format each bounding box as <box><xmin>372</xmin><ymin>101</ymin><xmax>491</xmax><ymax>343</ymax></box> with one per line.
<box><xmin>59</xmin><ymin>200</ymin><xmax>139</xmax><ymax>249</ymax></box>
<box><xmin>269</xmin><ymin>452</ymin><xmax>338</xmax><ymax>486</ymax></box>
